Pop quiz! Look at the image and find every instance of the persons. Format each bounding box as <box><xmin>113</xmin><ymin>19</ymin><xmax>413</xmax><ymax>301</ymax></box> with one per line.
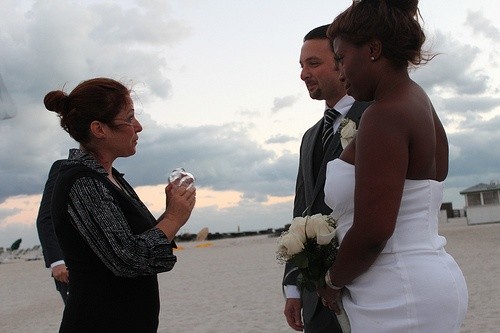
<box><xmin>280</xmin><ymin>21</ymin><xmax>369</xmax><ymax>333</ymax></box>
<box><xmin>35</xmin><ymin>158</ymin><xmax>76</xmax><ymax>309</ymax></box>
<box><xmin>318</xmin><ymin>0</ymin><xmax>470</xmax><ymax>333</ymax></box>
<box><xmin>42</xmin><ymin>77</ymin><xmax>197</xmax><ymax>333</ymax></box>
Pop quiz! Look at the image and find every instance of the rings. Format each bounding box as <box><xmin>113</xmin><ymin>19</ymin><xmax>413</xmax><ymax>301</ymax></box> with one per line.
<box><xmin>329</xmin><ymin>305</ymin><xmax>332</xmax><ymax>310</ymax></box>
<box><xmin>317</xmin><ymin>293</ymin><xmax>320</xmax><ymax>298</ymax></box>
<box><xmin>322</xmin><ymin>298</ymin><xmax>327</xmax><ymax>306</ymax></box>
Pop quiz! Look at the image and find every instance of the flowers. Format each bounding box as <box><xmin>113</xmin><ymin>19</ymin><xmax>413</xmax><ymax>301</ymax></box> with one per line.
<box><xmin>274</xmin><ymin>213</ymin><xmax>338</xmax><ymax>293</ymax></box>
<box><xmin>338</xmin><ymin>117</ymin><xmax>358</xmax><ymax>150</ymax></box>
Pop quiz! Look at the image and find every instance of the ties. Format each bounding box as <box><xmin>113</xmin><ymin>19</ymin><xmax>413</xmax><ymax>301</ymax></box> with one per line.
<box><xmin>320</xmin><ymin>108</ymin><xmax>339</xmax><ymax>153</ymax></box>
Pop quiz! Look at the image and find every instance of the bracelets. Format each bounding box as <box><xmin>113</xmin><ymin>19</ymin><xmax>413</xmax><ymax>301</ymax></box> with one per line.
<box><xmin>324</xmin><ymin>269</ymin><xmax>343</xmax><ymax>290</ymax></box>
